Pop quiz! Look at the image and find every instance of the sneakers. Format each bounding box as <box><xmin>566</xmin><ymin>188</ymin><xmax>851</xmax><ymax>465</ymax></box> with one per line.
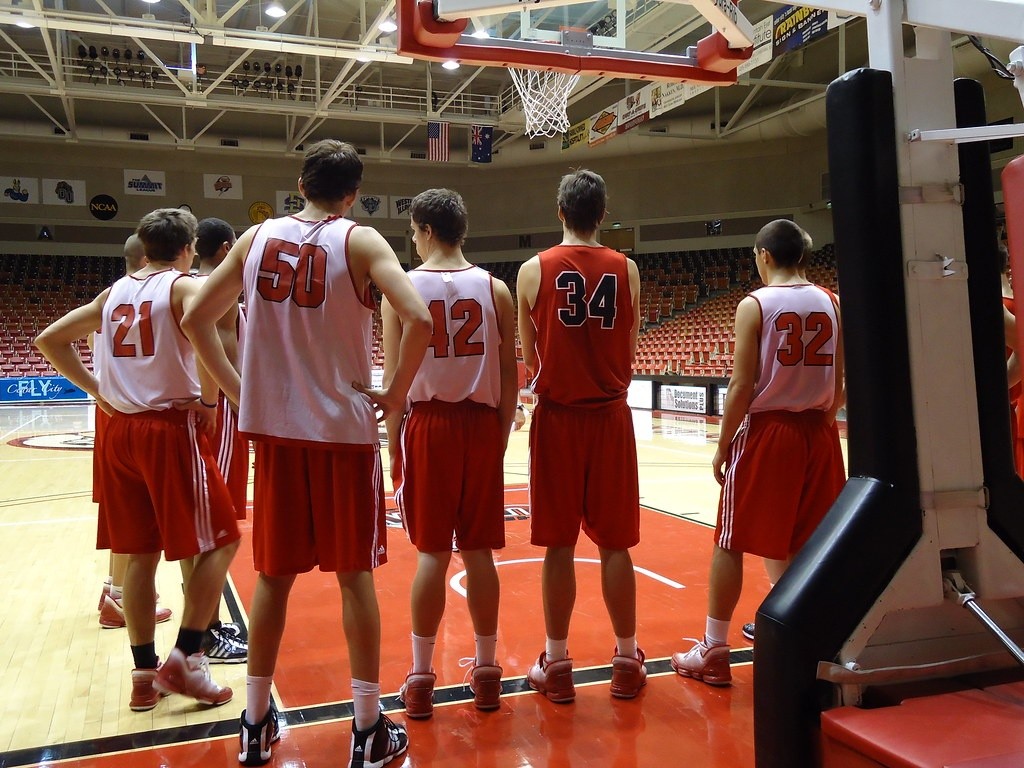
<box><xmin>459</xmin><ymin>657</ymin><xmax>503</xmax><ymax>709</ymax></box>
<box><xmin>610</xmin><ymin>645</ymin><xmax>647</xmax><ymax>697</ymax></box>
<box><xmin>99</xmin><ymin>595</ymin><xmax>172</xmax><ymax>628</ymax></box>
<box><xmin>348</xmin><ymin>705</ymin><xmax>409</xmax><ymax>768</ymax></box>
<box><xmin>152</xmin><ymin>648</ymin><xmax>233</xmax><ymax>704</ymax></box>
<box><xmin>528</xmin><ymin>649</ymin><xmax>576</xmax><ymax>701</ymax></box>
<box><xmin>98</xmin><ymin>581</ymin><xmax>160</xmax><ymax>610</ymax></box>
<box><xmin>130</xmin><ymin>660</ymin><xmax>171</xmax><ymax>710</ymax></box>
<box><xmin>205</xmin><ymin>621</ymin><xmax>247</xmax><ymax>663</ymax></box>
<box><xmin>395</xmin><ymin>663</ymin><xmax>436</xmax><ymax>717</ymax></box>
<box><xmin>671</xmin><ymin>633</ymin><xmax>732</xmax><ymax>684</ymax></box>
<box><xmin>239</xmin><ymin>705</ymin><xmax>280</xmax><ymax>765</ymax></box>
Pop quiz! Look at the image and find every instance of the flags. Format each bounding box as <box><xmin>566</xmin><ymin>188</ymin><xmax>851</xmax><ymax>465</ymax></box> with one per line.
<box><xmin>471</xmin><ymin>125</ymin><xmax>492</xmax><ymax>163</ymax></box>
<box><xmin>427</xmin><ymin>121</ymin><xmax>451</xmax><ymax>161</ymax></box>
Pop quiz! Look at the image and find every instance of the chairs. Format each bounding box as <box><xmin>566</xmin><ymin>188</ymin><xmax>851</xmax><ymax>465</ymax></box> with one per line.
<box><xmin>0</xmin><ymin>255</ymin><xmax>141</xmax><ymax>381</ymax></box>
<box><xmin>371</xmin><ymin>245</ymin><xmax>846</xmax><ymax>401</ymax></box>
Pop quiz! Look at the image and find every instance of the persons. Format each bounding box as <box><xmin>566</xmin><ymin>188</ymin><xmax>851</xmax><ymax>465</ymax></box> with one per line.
<box><xmin>512</xmin><ymin>392</ymin><xmax>526</xmax><ymax>431</ymax></box>
<box><xmin>512</xmin><ymin>167</ymin><xmax>647</xmax><ymax>702</ymax></box>
<box><xmin>381</xmin><ymin>187</ymin><xmax>518</xmax><ymax>720</ymax></box>
<box><xmin>179</xmin><ymin>140</ymin><xmax>434</xmax><ymax>768</ymax></box>
<box><xmin>672</xmin><ymin>221</ymin><xmax>844</xmax><ymax>686</ymax></box>
<box><xmin>993</xmin><ymin>233</ymin><xmax>1024</xmax><ymax>479</ymax></box>
<box><xmin>34</xmin><ymin>210</ymin><xmax>249</xmax><ymax>712</ymax></box>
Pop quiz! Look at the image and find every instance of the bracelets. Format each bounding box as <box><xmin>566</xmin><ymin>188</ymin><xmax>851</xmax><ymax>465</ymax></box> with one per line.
<box><xmin>199</xmin><ymin>397</ymin><xmax>219</xmax><ymax>409</ymax></box>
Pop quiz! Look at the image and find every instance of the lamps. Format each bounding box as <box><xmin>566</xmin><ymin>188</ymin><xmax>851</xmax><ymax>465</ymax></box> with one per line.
<box><xmin>590</xmin><ymin>9</ymin><xmax>616</xmax><ymax>37</ymax></box>
<box><xmin>378</xmin><ymin>13</ymin><xmax>397</xmax><ymax>34</ymax></box>
<box><xmin>77</xmin><ymin>43</ymin><xmax>160</xmax><ymax>88</ymax></box>
<box><xmin>231</xmin><ymin>61</ymin><xmax>303</xmax><ymax>100</ymax></box>
<box><xmin>265</xmin><ymin>0</ymin><xmax>286</xmax><ymax>18</ymax></box>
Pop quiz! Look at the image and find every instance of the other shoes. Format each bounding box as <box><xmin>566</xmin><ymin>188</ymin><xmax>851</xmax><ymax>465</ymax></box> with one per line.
<box><xmin>743</xmin><ymin>622</ymin><xmax>755</xmax><ymax>639</ymax></box>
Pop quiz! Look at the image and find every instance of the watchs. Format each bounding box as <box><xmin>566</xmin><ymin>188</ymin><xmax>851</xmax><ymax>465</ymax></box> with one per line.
<box><xmin>517</xmin><ymin>403</ymin><xmax>526</xmax><ymax>410</ymax></box>
